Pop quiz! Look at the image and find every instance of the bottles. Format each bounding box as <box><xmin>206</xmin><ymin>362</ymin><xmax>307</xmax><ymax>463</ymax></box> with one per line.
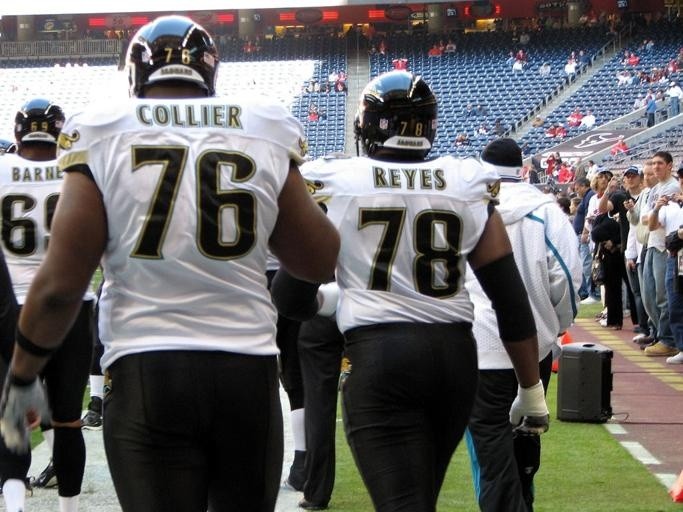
<box><xmin>597</xmin><ymin>165</ymin><xmax>642</xmax><ymax>178</ymax></box>
<box><xmin>480</xmin><ymin>139</ymin><xmax>525</xmax><ymax>180</ymax></box>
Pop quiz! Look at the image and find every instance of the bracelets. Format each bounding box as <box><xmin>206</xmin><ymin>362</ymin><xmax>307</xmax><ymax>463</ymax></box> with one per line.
<box><xmin>14</xmin><ymin>324</ymin><xmax>62</xmax><ymax>357</ymax></box>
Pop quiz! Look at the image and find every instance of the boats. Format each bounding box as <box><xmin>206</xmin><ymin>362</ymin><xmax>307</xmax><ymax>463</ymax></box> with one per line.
<box><xmin>555</xmin><ymin>341</ymin><xmax>614</xmax><ymax>424</ymax></box>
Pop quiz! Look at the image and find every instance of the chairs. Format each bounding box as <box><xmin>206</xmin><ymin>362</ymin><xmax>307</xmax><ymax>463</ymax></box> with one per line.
<box><xmin>596</xmin><ymin>306</ymin><xmax>618</xmax><ymax>331</ymax></box>
<box><xmin>632</xmin><ymin>324</ymin><xmax>654</xmax><ymax>349</ymax></box>
<box><xmin>81</xmin><ymin>397</ymin><xmax>105</xmax><ymax>431</ymax></box>
<box><xmin>645</xmin><ymin>343</ymin><xmax>677</xmax><ymax>357</ymax></box>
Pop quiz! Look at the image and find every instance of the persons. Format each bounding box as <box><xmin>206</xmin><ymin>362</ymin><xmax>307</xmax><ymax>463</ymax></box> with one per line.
<box><xmin>2</xmin><ymin>98</ymin><xmax>96</xmax><ymax>512</ymax></box>
<box><xmin>2</xmin><ymin>15</ymin><xmax>340</xmax><ymax>512</ymax></box>
<box><xmin>524</xmin><ymin>151</ymin><xmax>682</xmax><ymax>363</ymax></box>
<box><xmin>1</xmin><ymin>141</ymin><xmax>340</xmax><ymax>510</ymax></box>
<box><xmin>465</xmin><ymin>138</ymin><xmax>583</xmax><ymax>510</ymax></box>
<box><xmin>495</xmin><ymin>10</ymin><xmax>682</xmax><ymax>157</ymax></box>
<box><xmin>243</xmin><ymin>23</ymin><xmax>457</xmax><ymax>122</ymax></box>
<box><xmin>271</xmin><ymin>70</ymin><xmax>550</xmax><ymax>512</ymax></box>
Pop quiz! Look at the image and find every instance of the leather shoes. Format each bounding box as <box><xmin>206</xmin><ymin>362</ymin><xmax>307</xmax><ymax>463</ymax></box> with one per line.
<box><xmin>505</xmin><ymin>381</ymin><xmax>549</xmax><ymax>436</ymax></box>
<box><xmin>318</xmin><ymin>283</ymin><xmax>339</xmax><ymax>320</ymax></box>
<box><xmin>1</xmin><ymin>369</ymin><xmax>48</xmax><ymax>451</ymax></box>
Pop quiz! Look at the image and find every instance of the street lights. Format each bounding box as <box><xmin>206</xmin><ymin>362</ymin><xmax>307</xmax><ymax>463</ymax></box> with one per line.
<box><xmin>590</xmin><ymin>242</ymin><xmax>606</xmax><ymax>289</ymax></box>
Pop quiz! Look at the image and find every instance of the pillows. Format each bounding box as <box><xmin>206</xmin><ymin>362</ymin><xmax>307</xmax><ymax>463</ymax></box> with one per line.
<box><xmin>0</xmin><ymin>139</ymin><xmax>16</xmax><ymax>153</ymax></box>
<box><xmin>352</xmin><ymin>66</ymin><xmax>440</xmax><ymax>155</ymax></box>
<box><xmin>16</xmin><ymin>99</ymin><xmax>68</xmax><ymax>149</ymax></box>
<box><xmin>127</xmin><ymin>15</ymin><xmax>222</xmax><ymax>100</ymax></box>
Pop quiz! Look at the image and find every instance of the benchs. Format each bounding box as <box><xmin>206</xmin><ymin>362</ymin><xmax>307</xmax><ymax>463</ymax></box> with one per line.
<box><xmin>0</xmin><ymin>35</ymin><xmax>346</xmax><ymax>160</ymax></box>
<box><xmin>370</xmin><ymin>19</ymin><xmax>683</xmax><ymax>176</ymax></box>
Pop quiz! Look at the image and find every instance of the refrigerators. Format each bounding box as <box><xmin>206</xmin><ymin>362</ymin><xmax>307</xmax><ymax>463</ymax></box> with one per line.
<box><xmin>667</xmin><ymin>352</ymin><xmax>682</xmax><ymax>364</ymax></box>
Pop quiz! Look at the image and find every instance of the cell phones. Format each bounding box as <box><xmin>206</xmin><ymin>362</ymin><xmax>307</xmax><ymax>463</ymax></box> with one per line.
<box><xmin>664</xmin><ymin>194</ymin><xmax>674</xmax><ymax>201</ymax></box>
<box><xmin>616</xmin><ymin>177</ymin><xmax>623</xmax><ymax>184</ymax></box>
